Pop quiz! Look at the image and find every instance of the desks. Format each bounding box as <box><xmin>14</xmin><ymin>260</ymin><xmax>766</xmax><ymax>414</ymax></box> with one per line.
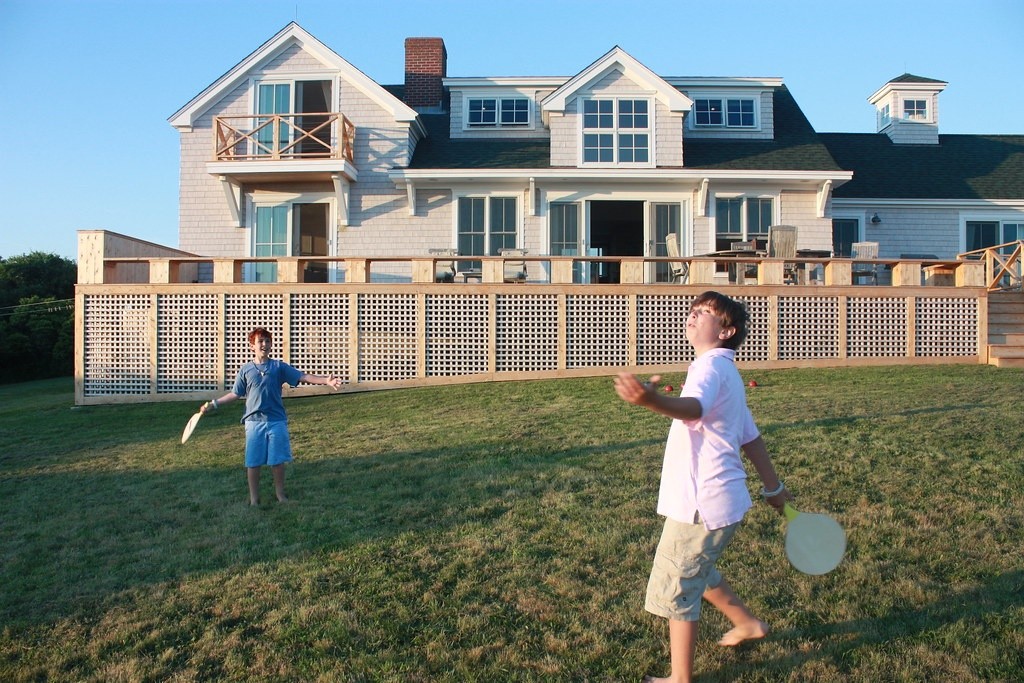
<box><xmin>688</xmin><ymin>249</ymin><xmax>767</xmax><ymax>284</ymax></box>
<box><xmin>797</xmin><ymin>249</ymin><xmax>831</xmax><ymax>282</ymax></box>
<box><xmin>462</xmin><ymin>272</ymin><xmax>482</xmax><ymax>283</ymax></box>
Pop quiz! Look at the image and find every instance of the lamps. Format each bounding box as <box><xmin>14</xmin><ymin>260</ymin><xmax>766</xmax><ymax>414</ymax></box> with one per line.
<box><xmin>872</xmin><ymin>212</ymin><xmax>882</xmax><ymax>224</ymax></box>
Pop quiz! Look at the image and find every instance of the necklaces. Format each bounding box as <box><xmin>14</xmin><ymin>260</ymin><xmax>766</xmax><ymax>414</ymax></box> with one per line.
<box><xmin>252</xmin><ymin>357</ymin><xmax>270</xmax><ymax>376</ymax></box>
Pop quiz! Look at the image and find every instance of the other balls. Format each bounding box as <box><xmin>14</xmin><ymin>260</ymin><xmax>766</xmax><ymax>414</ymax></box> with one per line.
<box><xmin>750</xmin><ymin>380</ymin><xmax>756</xmax><ymax>387</ymax></box>
<box><xmin>665</xmin><ymin>385</ymin><xmax>673</xmax><ymax>392</ymax></box>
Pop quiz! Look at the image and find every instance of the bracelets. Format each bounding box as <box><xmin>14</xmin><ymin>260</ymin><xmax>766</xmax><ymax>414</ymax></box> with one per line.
<box><xmin>212</xmin><ymin>399</ymin><xmax>219</xmax><ymax>410</ymax></box>
<box><xmin>761</xmin><ymin>481</ymin><xmax>784</xmax><ymax>497</ymax></box>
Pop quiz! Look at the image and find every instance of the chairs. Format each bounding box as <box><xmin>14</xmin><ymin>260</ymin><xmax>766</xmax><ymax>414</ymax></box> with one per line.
<box><xmin>665</xmin><ymin>235</ymin><xmax>690</xmax><ymax>284</ymax></box>
<box><xmin>729</xmin><ymin>238</ymin><xmax>758</xmax><ymax>284</ymax></box>
<box><xmin>427</xmin><ymin>248</ymin><xmax>458</xmax><ymax>283</ymax></box>
<box><xmin>767</xmin><ymin>224</ymin><xmax>799</xmax><ymax>286</ymax></box>
<box><xmin>497</xmin><ymin>247</ymin><xmax>529</xmax><ymax>283</ymax></box>
<box><xmin>850</xmin><ymin>241</ymin><xmax>880</xmax><ymax>287</ymax></box>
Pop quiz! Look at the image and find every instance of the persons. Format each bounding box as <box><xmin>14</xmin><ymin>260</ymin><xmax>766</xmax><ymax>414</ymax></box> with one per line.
<box><xmin>613</xmin><ymin>290</ymin><xmax>786</xmax><ymax>683</ymax></box>
<box><xmin>200</xmin><ymin>328</ymin><xmax>342</xmax><ymax>506</ymax></box>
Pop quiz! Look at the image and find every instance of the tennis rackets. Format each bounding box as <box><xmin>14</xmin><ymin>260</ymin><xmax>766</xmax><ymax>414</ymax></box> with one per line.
<box><xmin>178</xmin><ymin>403</ymin><xmax>213</xmax><ymax>445</ymax></box>
<box><xmin>785</xmin><ymin>504</ymin><xmax>848</xmax><ymax>575</ymax></box>
